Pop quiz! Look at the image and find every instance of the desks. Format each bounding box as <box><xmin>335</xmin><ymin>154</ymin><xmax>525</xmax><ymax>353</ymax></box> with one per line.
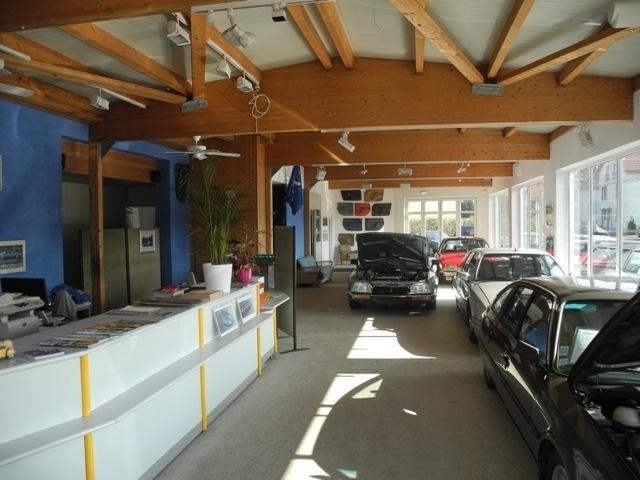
<box><xmin>76</xmin><ymin>300</ymin><xmax>94</xmax><ymax>318</ymax></box>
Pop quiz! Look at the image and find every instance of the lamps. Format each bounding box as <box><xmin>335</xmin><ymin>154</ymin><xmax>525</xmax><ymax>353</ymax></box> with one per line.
<box><xmin>165</xmin><ymin>11</ymin><xmax>192</xmax><ymax>47</ymax></box>
<box><xmin>396</xmin><ymin>163</ymin><xmax>413</xmax><ymax>177</ymax></box>
<box><xmin>336</xmin><ymin>130</ymin><xmax>356</xmax><ymax>154</ymax></box>
<box><xmin>455</xmin><ymin>162</ymin><xmax>466</xmax><ymax>175</ymax></box>
<box><xmin>313</xmin><ymin>167</ymin><xmax>327</xmax><ymax>181</ymax></box>
<box><xmin>235</xmin><ymin>71</ymin><xmax>255</xmax><ymax>94</ymax></box>
<box><xmin>230</xmin><ymin>24</ymin><xmax>258</xmax><ymax>50</ymax></box>
<box><xmin>214</xmin><ymin>55</ymin><xmax>232</xmax><ymax>79</ymax></box>
<box><xmin>357</xmin><ymin>163</ymin><xmax>369</xmax><ymax>176</ymax></box>
<box><xmin>88</xmin><ymin>89</ymin><xmax>110</xmax><ymax>112</ymax></box>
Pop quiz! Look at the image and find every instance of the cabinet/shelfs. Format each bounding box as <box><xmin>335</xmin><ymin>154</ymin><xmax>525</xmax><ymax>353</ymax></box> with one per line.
<box><xmin>0</xmin><ymin>275</ymin><xmax>291</xmax><ymax>480</ymax></box>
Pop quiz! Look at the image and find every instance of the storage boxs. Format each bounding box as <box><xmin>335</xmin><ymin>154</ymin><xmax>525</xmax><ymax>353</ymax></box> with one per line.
<box><xmin>124</xmin><ymin>205</ymin><xmax>157</xmax><ymax>229</ymax></box>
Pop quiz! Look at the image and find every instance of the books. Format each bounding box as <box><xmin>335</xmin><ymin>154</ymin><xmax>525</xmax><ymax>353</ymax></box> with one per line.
<box><xmin>152</xmin><ymin>287</ymin><xmax>184</xmax><ymax>299</ymax></box>
<box><xmin>22</xmin><ymin>347</ymin><xmax>64</xmax><ymax>361</ymax></box>
<box><xmin>181</xmin><ymin>290</ymin><xmax>223</xmax><ymax>301</ymax></box>
<box><xmin>41</xmin><ymin>321</ymin><xmax>159</xmax><ymax>349</ymax></box>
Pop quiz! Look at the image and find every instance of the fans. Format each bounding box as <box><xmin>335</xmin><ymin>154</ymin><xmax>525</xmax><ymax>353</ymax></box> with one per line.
<box><xmin>163</xmin><ymin>136</ymin><xmax>241</xmax><ymax>162</ymax></box>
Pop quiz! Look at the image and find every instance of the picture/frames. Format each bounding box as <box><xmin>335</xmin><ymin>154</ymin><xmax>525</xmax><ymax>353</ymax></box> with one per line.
<box><xmin>0</xmin><ymin>239</ymin><xmax>28</xmax><ymax>275</ymax></box>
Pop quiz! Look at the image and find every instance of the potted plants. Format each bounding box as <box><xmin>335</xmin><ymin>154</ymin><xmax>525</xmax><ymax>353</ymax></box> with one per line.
<box><xmin>168</xmin><ymin>160</ymin><xmax>256</xmax><ymax>294</ymax></box>
<box><xmin>227</xmin><ymin>216</ymin><xmax>273</xmax><ymax>285</ymax></box>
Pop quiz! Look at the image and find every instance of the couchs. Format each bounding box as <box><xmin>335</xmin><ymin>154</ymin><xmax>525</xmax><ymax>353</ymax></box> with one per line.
<box><xmin>296</xmin><ymin>255</ymin><xmax>335</xmax><ymax>289</ymax></box>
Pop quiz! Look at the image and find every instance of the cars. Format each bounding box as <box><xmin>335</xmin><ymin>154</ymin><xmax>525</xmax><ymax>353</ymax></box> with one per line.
<box><xmin>545</xmin><ymin>233</ymin><xmax>640</xmax><ymax>276</ymax></box>
<box><xmin>478</xmin><ymin>277</ymin><xmax>639</xmax><ymax>479</ymax></box>
<box><xmin>345</xmin><ymin>228</ymin><xmax>440</xmax><ymax>311</ymax></box>
<box><xmin>454</xmin><ymin>249</ymin><xmax>575</xmax><ymax>340</ymax></box>
<box><xmin>437</xmin><ymin>237</ymin><xmax>491</xmax><ymax>281</ymax></box>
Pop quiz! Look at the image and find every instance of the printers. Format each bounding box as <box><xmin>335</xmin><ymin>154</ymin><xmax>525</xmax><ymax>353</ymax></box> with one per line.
<box><xmin>0</xmin><ymin>292</ymin><xmax>45</xmax><ymax>340</ymax></box>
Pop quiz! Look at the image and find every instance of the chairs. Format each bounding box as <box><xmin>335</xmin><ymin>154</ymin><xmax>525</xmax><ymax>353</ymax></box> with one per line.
<box><xmin>512</xmin><ymin>296</ymin><xmax>618</xmax><ymax>347</ymax></box>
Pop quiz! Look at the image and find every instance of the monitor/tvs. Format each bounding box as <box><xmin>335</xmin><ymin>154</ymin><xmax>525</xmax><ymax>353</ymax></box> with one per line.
<box><xmin>0</xmin><ymin>276</ymin><xmax>51</xmax><ymax>314</ymax></box>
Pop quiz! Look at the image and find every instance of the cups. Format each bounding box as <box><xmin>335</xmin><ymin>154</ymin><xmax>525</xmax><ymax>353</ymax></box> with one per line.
<box><xmin>239</xmin><ymin>265</ymin><xmax>252</xmax><ymax>284</ymax></box>
